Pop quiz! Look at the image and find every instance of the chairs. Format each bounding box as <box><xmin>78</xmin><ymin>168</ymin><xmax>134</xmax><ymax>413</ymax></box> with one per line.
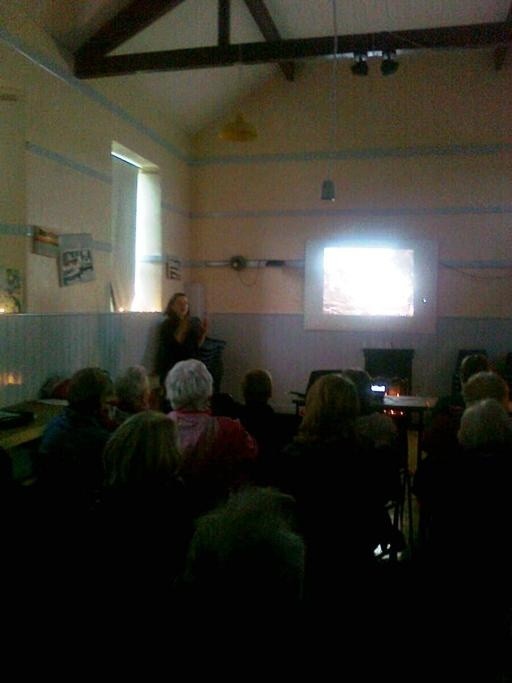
<box><xmin>451</xmin><ymin>348</ymin><xmax>492</xmax><ymax>396</ymax></box>
<box><xmin>292</xmin><ymin>367</ymin><xmax>342</xmax><ymax>412</ymax></box>
<box><xmin>364</xmin><ymin>346</ymin><xmax>416</xmax><ymax>397</ymax></box>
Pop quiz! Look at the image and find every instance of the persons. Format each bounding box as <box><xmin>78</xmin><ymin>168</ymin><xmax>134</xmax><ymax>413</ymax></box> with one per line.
<box><xmin>0</xmin><ymin>352</ymin><xmax>512</xmax><ymax>683</ymax></box>
<box><xmin>147</xmin><ymin>291</ymin><xmax>209</xmax><ymax>403</ymax></box>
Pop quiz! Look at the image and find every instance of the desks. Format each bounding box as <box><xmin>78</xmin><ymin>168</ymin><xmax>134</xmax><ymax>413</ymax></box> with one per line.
<box><xmin>366</xmin><ymin>391</ymin><xmax>442</xmax><ymax>479</ymax></box>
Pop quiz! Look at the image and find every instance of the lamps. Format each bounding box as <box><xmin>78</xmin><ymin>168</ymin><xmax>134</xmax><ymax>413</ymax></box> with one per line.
<box><xmin>351</xmin><ymin>29</ymin><xmax>401</xmax><ymax>77</ymax></box>
